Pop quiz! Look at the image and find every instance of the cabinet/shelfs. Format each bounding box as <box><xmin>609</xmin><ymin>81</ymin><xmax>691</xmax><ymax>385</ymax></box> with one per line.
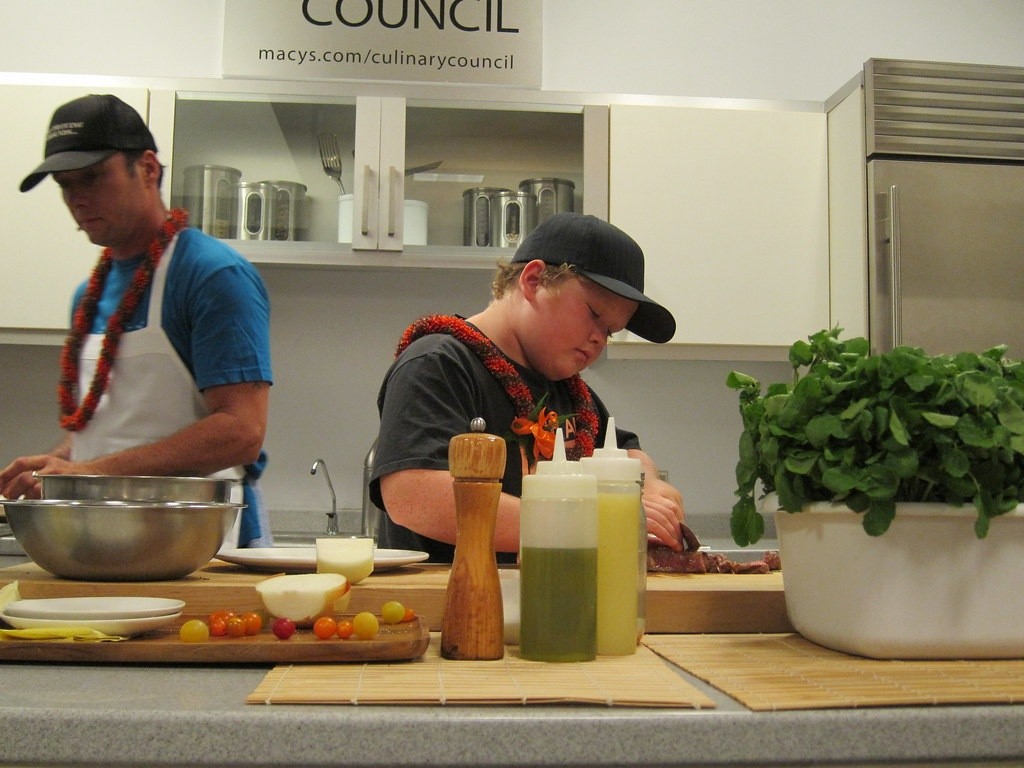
<box><xmin>0</xmin><ymin>65</ymin><xmax>830</xmax><ymax>364</ymax></box>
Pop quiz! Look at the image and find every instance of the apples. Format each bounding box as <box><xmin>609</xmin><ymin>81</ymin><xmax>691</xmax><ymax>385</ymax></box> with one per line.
<box><xmin>316</xmin><ymin>538</ymin><xmax>375</xmax><ymax>585</ymax></box>
<box><xmin>254</xmin><ymin>573</ymin><xmax>351</xmax><ymax>629</ymax></box>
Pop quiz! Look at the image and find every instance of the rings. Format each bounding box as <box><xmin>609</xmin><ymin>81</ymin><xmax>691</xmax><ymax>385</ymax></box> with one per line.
<box><xmin>32</xmin><ymin>470</ymin><xmax>40</xmax><ymax>481</ymax></box>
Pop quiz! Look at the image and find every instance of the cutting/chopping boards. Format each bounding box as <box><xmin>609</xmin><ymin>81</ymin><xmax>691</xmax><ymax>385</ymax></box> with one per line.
<box><xmin>0</xmin><ymin>608</ymin><xmax>433</xmax><ymax>664</ymax></box>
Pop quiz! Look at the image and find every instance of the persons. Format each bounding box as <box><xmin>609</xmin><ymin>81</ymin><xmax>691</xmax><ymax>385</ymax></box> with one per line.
<box><xmin>0</xmin><ymin>94</ymin><xmax>272</xmax><ymax>552</ymax></box>
<box><xmin>368</xmin><ymin>214</ymin><xmax>685</xmax><ymax>562</ymax></box>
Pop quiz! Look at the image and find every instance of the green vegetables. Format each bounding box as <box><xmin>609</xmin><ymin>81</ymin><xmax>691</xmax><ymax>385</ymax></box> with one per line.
<box><xmin>730</xmin><ymin>322</ymin><xmax>1024</xmax><ymax>546</ymax></box>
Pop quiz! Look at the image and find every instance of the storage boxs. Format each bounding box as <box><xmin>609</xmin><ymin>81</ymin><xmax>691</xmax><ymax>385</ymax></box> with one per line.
<box><xmin>774</xmin><ymin>502</ymin><xmax>1024</xmax><ymax>658</ymax></box>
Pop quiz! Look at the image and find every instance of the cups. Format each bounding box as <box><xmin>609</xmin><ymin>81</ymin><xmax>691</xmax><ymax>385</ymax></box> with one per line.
<box><xmin>633</xmin><ymin>474</ymin><xmax>647</xmax><ymax>642</ymax></box>
<box><xmin>519</xmin><ymin>426</ymin><xmax>602</xmax><ymax>661</ymax></box>
<box><xmin>579</xmin><ymin>412</ymin><xmax>642</xmax><ymax>657</ymax></box>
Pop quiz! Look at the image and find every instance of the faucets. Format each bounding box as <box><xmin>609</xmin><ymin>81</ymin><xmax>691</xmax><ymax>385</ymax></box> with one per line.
<box><xmin>310</xmin><ymin>459</ymin><xmax>339</xmax><ymax>535</ymax></box>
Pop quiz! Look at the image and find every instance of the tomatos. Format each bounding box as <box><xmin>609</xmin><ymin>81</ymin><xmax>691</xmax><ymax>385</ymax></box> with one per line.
<box><xmin>314</xmin><ymin>611</ymin><xmax>379</xmax><ymax>639</ymax></box>
<box><xmin>382</xmin><ymin>600</ymin><xmax>415</xmax><ymax>623</ymax></box>
<box><xmin>273</xmin><ymin>618</ymin><xmax>295</xmax><ymax>640</ymax></box>
<box><xmin>181</xmin><ymin>612</ymin><xmax>260</xmax><ymax>641</ymax></box>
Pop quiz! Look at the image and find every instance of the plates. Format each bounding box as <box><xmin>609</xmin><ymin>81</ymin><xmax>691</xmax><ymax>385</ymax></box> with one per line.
<box><xmin>1</xmin><ymin>595</ymin><xmax>184</xmax><ymax>636</ymax></box>
<box><xmin>212</xmin><ymin>545</ymin><xmax>430</xmax><ymax>574</ymax></box>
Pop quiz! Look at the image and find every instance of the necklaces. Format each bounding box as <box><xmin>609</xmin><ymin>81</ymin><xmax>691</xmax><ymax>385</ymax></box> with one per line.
<box><xmin>392</xmin><ymin>314</ymin><xmax>599</xmax><ymax>463</ymax></box>
<box><xmin>58</xmin><ymin>205</ymin><xmax>189</xmax><ymax>431</ymax></box>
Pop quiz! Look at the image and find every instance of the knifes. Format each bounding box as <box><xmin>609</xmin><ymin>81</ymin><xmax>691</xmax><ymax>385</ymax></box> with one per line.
<box><xmin>680</xmin><ymin>522</ymin><xmax>701</xmax><ymax>553</ymax></box>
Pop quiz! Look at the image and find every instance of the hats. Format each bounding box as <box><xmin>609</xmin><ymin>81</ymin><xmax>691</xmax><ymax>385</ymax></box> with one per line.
<box><xmin>20</xmin><ymin>94</ymin><xmax>157</xmax><ymax>192</ymax></box>
<box><xmin>510</xmin><ymin>213</ymin><xmax>676</xmax><ymax>343</ymax></box>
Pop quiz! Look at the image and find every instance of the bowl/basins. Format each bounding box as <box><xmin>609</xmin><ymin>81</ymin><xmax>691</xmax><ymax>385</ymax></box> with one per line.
<box><xmin>33</xmin><ymin>473</ymin><xmax>242</xmax><ymax>500</ymax></box>
<box><xmin>0</xmin><ymin>496</ymin><xmax>249</xmax><ymax>580</ymax></box>
